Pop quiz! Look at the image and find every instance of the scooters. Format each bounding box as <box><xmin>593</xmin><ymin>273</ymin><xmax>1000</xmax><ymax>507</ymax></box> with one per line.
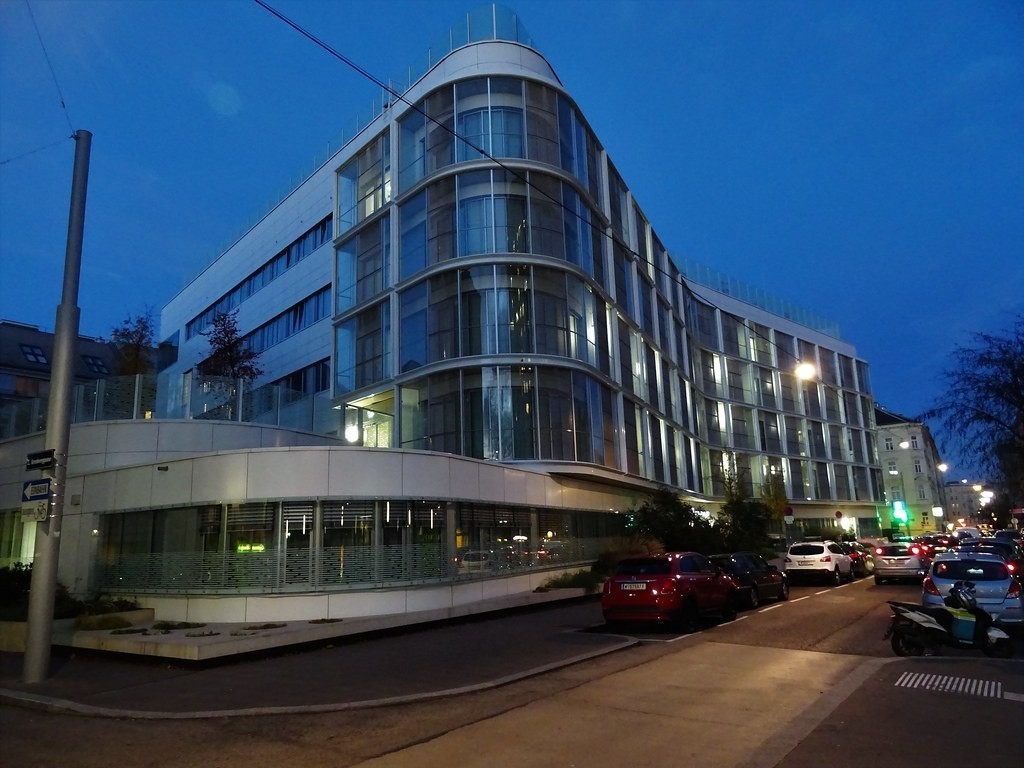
<box><xmin>884</xmin><ymin>577</ymin><xmax>1016</xmax><ymax>661</ymax></box>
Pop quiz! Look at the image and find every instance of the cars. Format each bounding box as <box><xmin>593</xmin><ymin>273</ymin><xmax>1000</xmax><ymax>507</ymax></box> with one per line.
<box><xmin>840</xmin><ymin>513</ymin><xmax>1023</xmax><ymax>630</ymax></box>
<box><xmin>704</xmin><ymin>554</ymin><xmax>790</xmax><ymax>609</ymax></box>
<box><xmin>784</xmin><ymin>536</ymin><xmax>855</xmax><ymax>590</ymax></box>
<box><xmin>596</xmin><ymin>553</ymin><xmax>745</xmax><ymax>631</ymax></box>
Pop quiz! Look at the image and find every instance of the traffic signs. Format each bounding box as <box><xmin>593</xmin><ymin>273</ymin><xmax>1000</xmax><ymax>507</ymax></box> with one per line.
<box><xmin>19</xmin><ymin>448</ymin><xmax>59</xmax><ymax>523</ymax></box>
